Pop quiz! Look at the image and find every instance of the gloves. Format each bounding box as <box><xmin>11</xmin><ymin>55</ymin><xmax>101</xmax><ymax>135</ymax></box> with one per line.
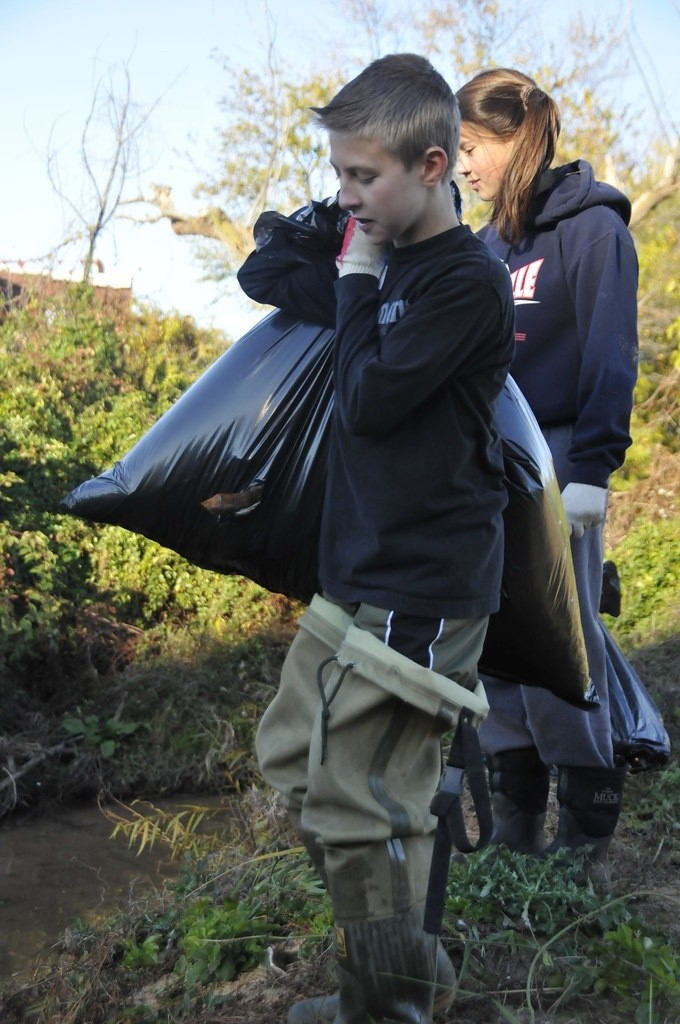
<box><xmin>336</xmin><ymin>217</ymin><xmax>389</xmax><ymax>279</ymax></box>
<box><xmin>561</xmin><ymin>482</ymin><xmax>608</xmax><ymax>538</ymax></box>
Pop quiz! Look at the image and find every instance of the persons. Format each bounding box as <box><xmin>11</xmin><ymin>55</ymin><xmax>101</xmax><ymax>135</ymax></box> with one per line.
<box><xmin>236</xmin><ymin>53</ymin><xmax>516</xmax><ymax>1023</ymax></box>
<box><xmin>455</xmin><ymin>70</ymin><xmax>639</xmax><ymax>912</ymax></box>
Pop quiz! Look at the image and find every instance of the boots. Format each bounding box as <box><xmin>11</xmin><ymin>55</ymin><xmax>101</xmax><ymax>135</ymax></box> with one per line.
<box><xmin>288</xmin><ymin>939</ymin><xmax>457</xmax><ymax>1024</ymax></box>
<box><xmin>538</xmin><ymin>757</ymin><xmax>630</xmax><ymax>901</ymax></box>
<box><xmin>450</xmin><ymin>747</ymin><xmax>549</xmax><ymax>864</ymax></box>
